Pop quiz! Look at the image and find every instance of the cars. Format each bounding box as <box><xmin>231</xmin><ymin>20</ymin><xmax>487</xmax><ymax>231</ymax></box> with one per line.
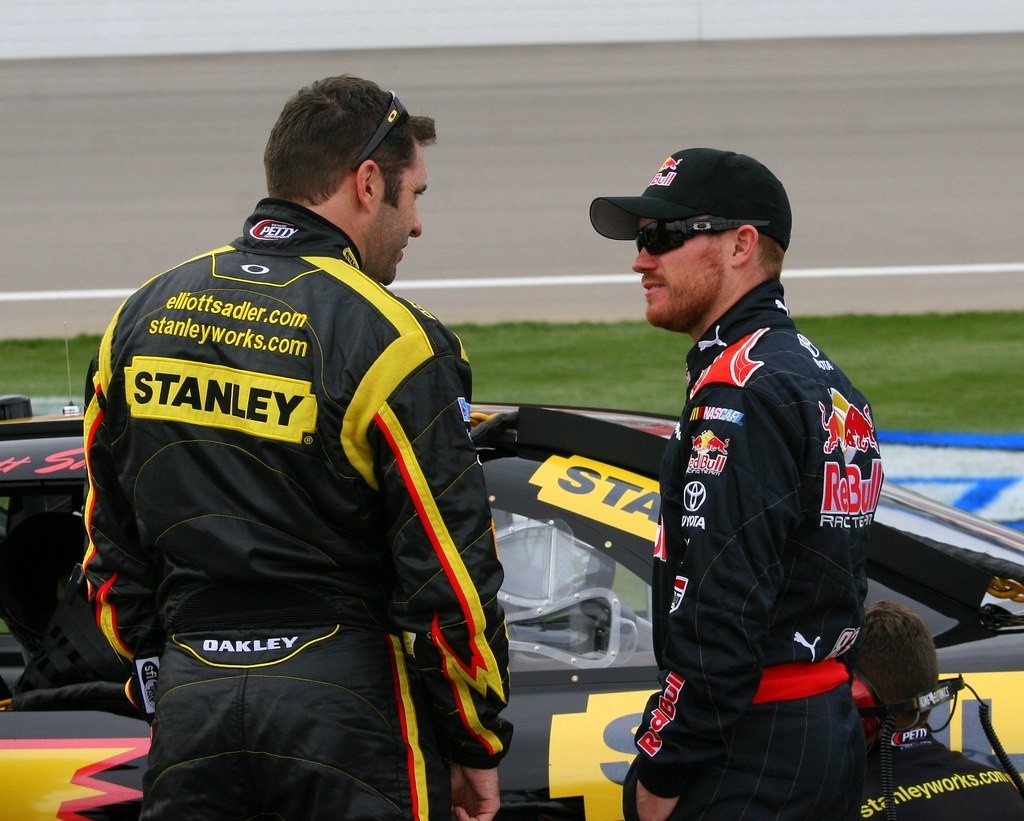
<box><xmin>0</xmin><ymin>391</ymin><xmax>1020</xmax><ymax>821</ymax></box>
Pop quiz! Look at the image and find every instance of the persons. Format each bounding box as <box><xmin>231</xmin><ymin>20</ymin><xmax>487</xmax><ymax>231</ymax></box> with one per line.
<box><xmin>589</xmin><ymin>147</ymin><xmax>885</xmax><ymax>821</ymax></box>
<box><xmin>78</xmin><ymin>76</ymin><xmax>514</xmax><ymax>821</ymax></box>
<box><xmin>863</xmin><ymin>602</ymin><xmax>1024</xmax><ymax>821</ymax></box>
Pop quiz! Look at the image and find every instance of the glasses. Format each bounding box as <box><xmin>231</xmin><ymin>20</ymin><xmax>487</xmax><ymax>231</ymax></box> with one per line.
<box><xmin>635</xmin><ymin>217</ymin><xmax>771</xmax><ymax>255</ymax></box>
<box><xmin>351</xmin><ymin>90</ymin><xmax>410</xmax><ymax>172</ymax></box>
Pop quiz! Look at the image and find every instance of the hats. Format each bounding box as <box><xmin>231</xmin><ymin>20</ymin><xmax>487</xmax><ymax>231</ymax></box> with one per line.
<box><xmin>589</xmin><ymin>148</ymin><xmax>792</xmax><ymax>252</ymax></box>
